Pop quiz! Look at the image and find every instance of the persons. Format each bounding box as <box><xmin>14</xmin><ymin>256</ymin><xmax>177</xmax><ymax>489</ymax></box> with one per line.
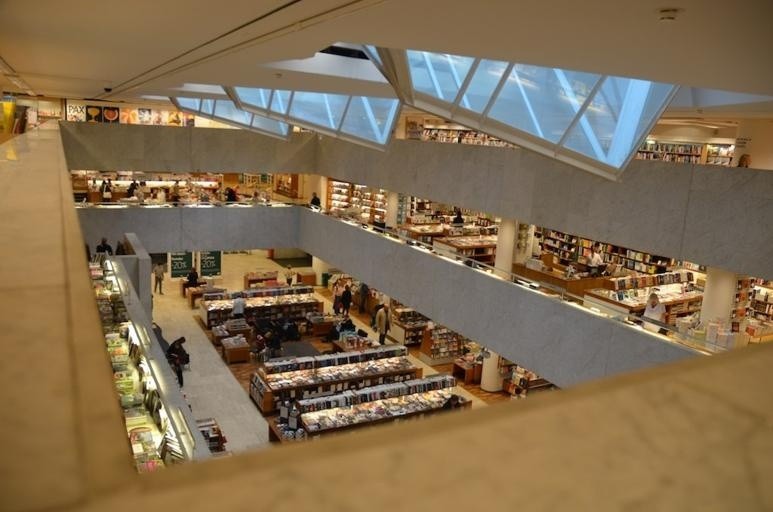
<box><xmin>185</xmin><ymin>267</ymin><xmax>198</xmax><ymax>288</ymax></box>
<box><xmin>428</xmin><ymin>132</ymin><xmax>466</xmax><ymax>144</ymax></box>
<box><xmin>166</xmin><ymin>336</ymin><xmax>189</xmax><ymax>386</ymax></box>
<box><xmin>128</xmin><ymin>178</ymin><xmax>139</xmax><ymax>196</ymax></box>
<box><xmin>250</xmin><ymin>282</ymin><xmax>393</xmax><ymax>358</ymax></box>
<box><xmin>311</xmin><ymin>192</ymin><xmax>320</xmax><ymax>208</ymax></box>
<box><xmin>100</xmin><ymin>181</ymin><xmax>112</xmax><ymax>202</ymax></box>
<box><xmin>737</xmin><ymin>154</ymin><xmax>752</xmax><ymax>167</ymax></box>
<box><xmin>223</xmin><ymin>184</ymin><xmax>263</xmax><ymax>204</ymax></box>
<box><xmin>84</xmin><ymin>238</ymin><xmax>125</xmax><ymax>261</ymax></box>
<box><xmin>644</xmin><ymin>293</ymin><xmax>666</xmax><ymax>334</ymax></box>
<box><xmin>284</xmin><ymin>265</ymin><xmax>297</xmax><ymax>286</ymax></box>
<box><xmin>586</xmin><ymin>246</ymin><xmax>603</xmax><ymax>267</ymax></box>
<box><xmin>153</xmin><ymin>262</ymin><xmax>164</xmax><ymax>295</ymax></box>
<box><xmin>453</xmin><ymin>211</ymin><xmax>463</xmax><ymax>227</ymax></box>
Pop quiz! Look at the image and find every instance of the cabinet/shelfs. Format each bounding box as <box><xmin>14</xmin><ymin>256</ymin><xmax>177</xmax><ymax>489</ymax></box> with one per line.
<box><xmin>188</xmin><ymin>276</ymin><xmax>551</xmax><ymax>444</ymax></box>
<box><xmin>321</xmin><ymin>112</ymin><xmax>771</xmax><ymax>351</ymax></box>
